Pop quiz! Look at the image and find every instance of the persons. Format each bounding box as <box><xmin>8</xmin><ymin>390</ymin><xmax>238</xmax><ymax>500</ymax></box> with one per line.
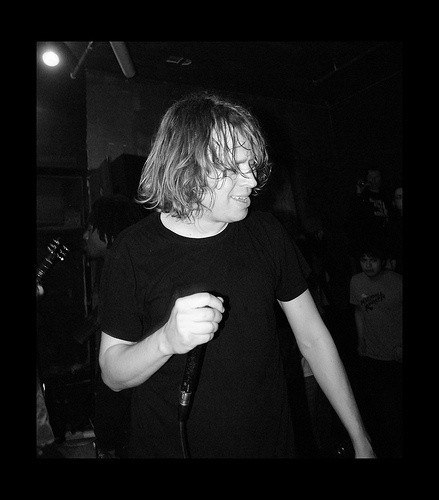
<box><xmin>99</xmin><ymin>92</ymin><xmax>377</xmax><ymax>459</ymax></box>
<box><xmin>37</xmin><ymin>161</ymin><xmax>403</xmax><ymax>457</ymax></box>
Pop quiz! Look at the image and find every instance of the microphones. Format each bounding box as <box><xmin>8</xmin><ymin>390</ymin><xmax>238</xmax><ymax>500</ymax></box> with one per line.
<box><xmin>36</xmin><ymin>230</ymin><xmax>80</xmax><ymax>283</ymax></box>
<box><xmin>177</xmin><ymin>290</ymin><xmax>214</xmax><ymax>421</ymax></box>
